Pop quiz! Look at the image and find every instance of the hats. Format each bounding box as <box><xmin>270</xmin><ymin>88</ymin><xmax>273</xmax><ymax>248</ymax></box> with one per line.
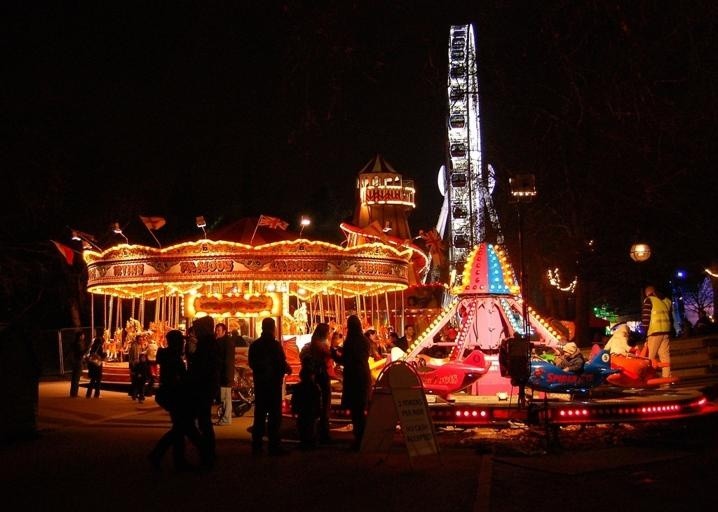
<box><xmin>191</xmin><ymin>316</ymin><xmax>214</xmax><ymax>335</ymax></box>
<box><xmin>165</xmin><ymin>330</ymin><xmax>188</xmax><ymax>340</ymax></box>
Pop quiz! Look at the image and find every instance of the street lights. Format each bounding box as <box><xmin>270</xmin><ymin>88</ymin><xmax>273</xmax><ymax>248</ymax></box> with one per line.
<box><xmin>509</xmin><ymin>173</ymin><xmax>537</xmax><ymax>336</ymax></box>
<box><xmin>630</xmin><ymin>243</ymin><xmax>651</xmax><ymax>305</ymax></box>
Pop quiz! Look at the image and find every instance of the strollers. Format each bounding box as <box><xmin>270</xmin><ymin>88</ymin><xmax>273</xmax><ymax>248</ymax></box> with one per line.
<box><xmin>217</xmin><ymin>368</ymin><xmax>255</xmax><ymax>417</ymax></box>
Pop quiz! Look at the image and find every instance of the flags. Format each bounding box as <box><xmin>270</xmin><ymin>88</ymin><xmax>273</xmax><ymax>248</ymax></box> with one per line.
<box><xmin>259</xmin><ymin>215</ymin><xmax>290</xmax><ymax>230</ymax></box>
<box><xmin>142</xmin><ymin>216</ymin><xmax>168</xmax><ymax>230</ymax></box>
<box><xmin>55</xmin><ymin>241</ymin><xmax>75</xmax><ymax>266</ymax></box>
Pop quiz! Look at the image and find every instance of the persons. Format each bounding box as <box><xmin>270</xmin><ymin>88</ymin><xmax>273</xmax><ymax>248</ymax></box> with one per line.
<box><xmin>232</xmin><ymin>330</ymin><xmax>247</xmax><ymax>347</ymax></box>
<box><xmin>603</xmin><ymin>285</ymin><xmax>671</xmax><ymax>389</ymax></box>
<box><xmin>298</xmin><ymin>323</ymin><xmax>330</xmax><ymax>440</ymax></box>
<box><xmin>397</xmin><ymin>325</ymin><xmax>415</xmax><ymax>353</ymax></box>
<box><xmin>556</xmin><ymin>341</ymin><xmax>584</xmax><ymax>372</ymax></box>
<box><xmin>364</xmin><ymin>330</ymin><xmax>375</xmax><ymax>340</ymax></box>
<box><xmin>68</xmin><ymin>315</ymin><xmax>227</xmax><ymax>473</ymax></box>
<box><xmin>330</xmin><ymin>315</ymin><xmax>372</xmax><ymax>453</ymax></box>
<box><xmin>247</xmin><ymin>317</ymin><xmax>292</xmax><ymax>457</ymax></box>
<box><xmin>291</xmin><ymin>366</ymin><xmax>323</xmax><ymax>445</ymax></box>
<box><xmin>214</xmin><ymin>323</ymin><xmax>234</xmax><ymax>426</ymax></box>
